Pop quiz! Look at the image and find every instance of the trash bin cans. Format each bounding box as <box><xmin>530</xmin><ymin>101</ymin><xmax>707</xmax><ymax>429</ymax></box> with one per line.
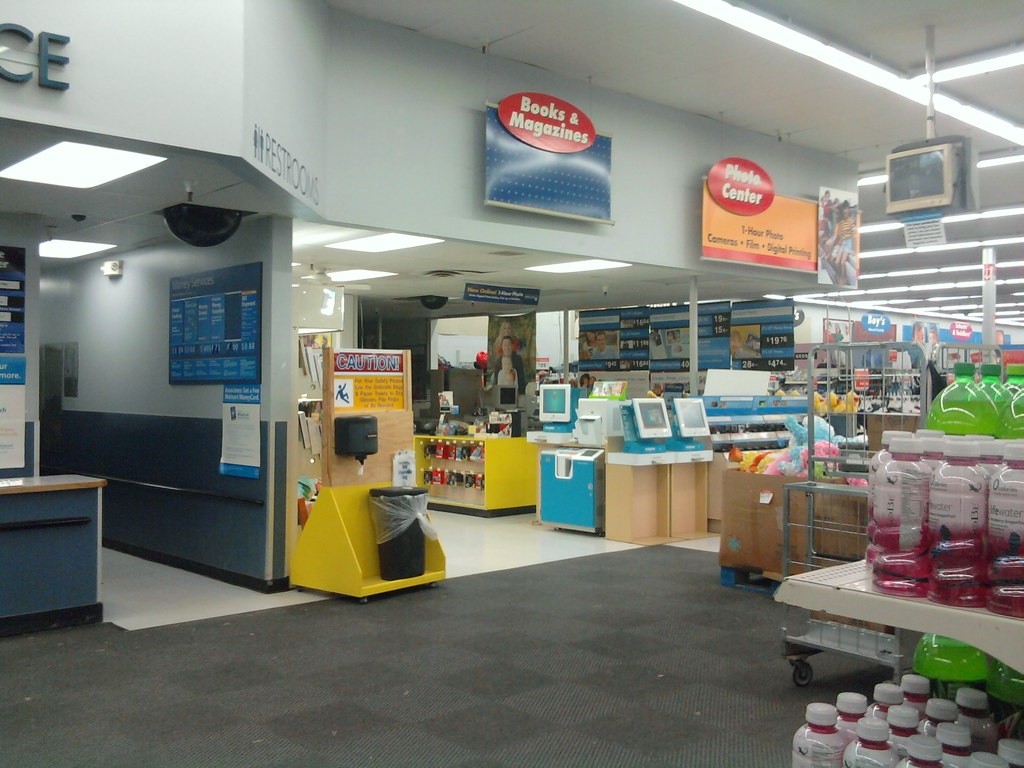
<box><xmin>369</xmin><ymin>486</ymin><xmax>428</xmax><ymax>581</ymax></box>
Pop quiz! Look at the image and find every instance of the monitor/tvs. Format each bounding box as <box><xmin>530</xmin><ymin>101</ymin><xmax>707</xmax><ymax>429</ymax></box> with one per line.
<box><xmin>886</xmin><ymin>143</ymin><xmax>963</xmax><ymax>215</ymax></box>
<box><xmin>664</xmin><ymin>397</ymin><xmax>711</xmax><ymax>451</ymax></box>
<box><xmin>495</xmin><ymin>385</ymin><xmax>518</xmax><ymax>408</ymax></box>
<box><xmin>619</xmin><ymin>398</ymin><xmax>672</xmax><ymax>454</ymax></box>
<box><xmin>539</xmin><ymin>384</ymin><xmax>580</xmax><ymax>433</ymax></box>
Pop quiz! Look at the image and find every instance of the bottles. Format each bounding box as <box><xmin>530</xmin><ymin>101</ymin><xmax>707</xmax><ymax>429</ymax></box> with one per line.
<box><xmin>790</xmin><ymin>360</ymin><xmax>1024</xmax><ymax>768</ymax></box>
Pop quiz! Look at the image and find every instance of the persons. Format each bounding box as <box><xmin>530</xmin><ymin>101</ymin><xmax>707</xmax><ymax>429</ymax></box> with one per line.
<box><xmin>494</xmin><ymin>321</ymin><xmax>527</xmax><ymax>394</ymax></box>
<box><xmin>730</xmin><ymin>330</ymin><xmax>761</xmax><ymax>358</ymax></box>
<box><xmin>299</xmin><ymin>401</ymin><xmax>323</xmax><ymax>419</ymax></box>
<box><xmin>583</xmin><ymin>330</ymin><xmax>618</xmax><ymax>358</ymax></box>
<box><xmin>579</xmin><ymin>373</ymin><xmax>596</xmax><ymax>388</ymax></box>
<box><xmin>646</xmin><ymin>384</ymin><xmax>663</xmax><ymax>398</ymax></box>
<box><xmin>650</xmin><ymin>329</ymin><xmax>689</xmax><ymax>358</ymax></box>
<box><xmin>820</xmin><ymin>191</ymin><xmax>857</xmax><ymax>285</ymax></box>
<box><xmin>302</xmin><ymin>335</ymin><xmax>330</xmax><ymax>349</ymax></box>
<box><xmin>825</xmin><ymin>321</ymin><xmax>851</xmax><ymax>343</ymax></box>
<box><xmin>912</xmin><ymin>322</ymin><xmax>939</xmax><ymax>347</ymax></box>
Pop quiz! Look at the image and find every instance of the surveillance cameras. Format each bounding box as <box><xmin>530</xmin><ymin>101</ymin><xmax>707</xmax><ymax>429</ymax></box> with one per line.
<box><xmin>71</xmin><ymin>214</ymin><xmax>86</xmax><ymax>222</ymax></box>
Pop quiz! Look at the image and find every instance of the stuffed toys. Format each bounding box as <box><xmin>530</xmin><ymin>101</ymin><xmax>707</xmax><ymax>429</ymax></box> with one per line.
<box><xmin>813</xmin><ymin>390</ymin><xmax>860</xmax><ymax>415</ymax></box>
<box><xmin>727</xmin><ymin>415</ymin><xmax>870</xmax><ymax>486</ymax></box>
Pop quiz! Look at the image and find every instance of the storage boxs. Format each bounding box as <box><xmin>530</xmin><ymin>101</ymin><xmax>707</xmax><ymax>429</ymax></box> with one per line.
<box><xmin>717</xmin><ymin>465</ymin><xmax>869</xmax><ymax>579</ymax></box>
